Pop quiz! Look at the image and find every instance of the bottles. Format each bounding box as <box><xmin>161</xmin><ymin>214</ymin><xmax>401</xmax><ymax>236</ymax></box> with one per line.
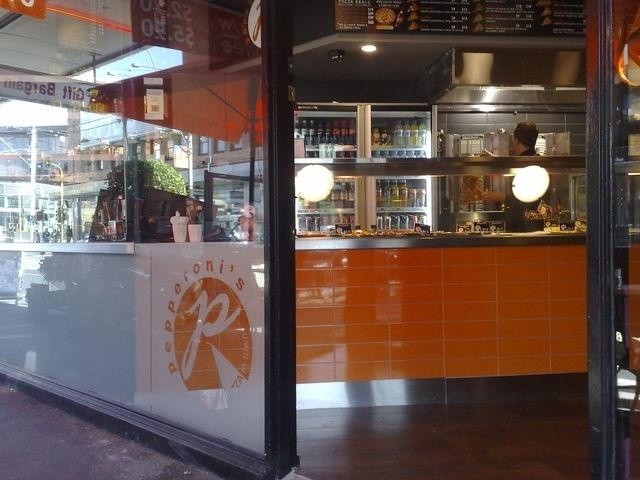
<box><xmin>330</xmin><ymin>215</ymin><xmax>341</xmax><ymax>226</ymax></box>
<box><xmin>326</xmin><ymin>120</ymin><xmax>333</xmax><ymax>143</ymax></box>
<box><xmin>419</xmin><ymin>120</ymin><xmax>426</xmax><ymax>145</ymax></box>
<box><xmin>306</xmin><ymin>217</ymin><xmax>315</xmax><ymax>231</ymax></box>
<box><xmin>348</xmin><ymin>120</ymin><xmax>356</xmax><ymax>145</ymax></box>
<box><xmin>384</xmin><ymin>216</ymin><xmax>392</xmax><ymax>229</ymax></box>
<box><xmin>403</xmin><ymin>120</ymin><xmax>411</xmax><ymax>146</ymax></box>
<box><xmin>411</xmin><ymin>119</ymin><xmax>419</xmax><ymax>145</ymax></box>
<box><xmin>321</xmin><ymin>214</ymin><xmax>330</xmax><ymax>226</ymax></box>
<box><xmin>333</xmin><ymin>120</ymin><xmax>341</xmax><ymax>145</ymax></box>
<box><xmin>340</xmin><ymin>120</ymin><xmax>348</xmax><ymax>145</ymax></box>
<box><xmin>318</xmin><ymin>120</ymin><xmax>325</xmax><ymax>143</ymax></box>
<box><xmin>395</xmin><ymin>120</ymin><xmax>403</xmax><ymax>146</ymax></box>
<box><xmin>390</xmin><ymin>179</ymin><xmax>399</xmax><ymax>207</ymax></box>
<box><xmin>401</xmin><ymin>216</ymin><xmax>410</xmax><ymax>229</ymax></box>
<box><xmin>409</xmin><ymin>215</ymin><xmax>419</xmax><ymax>229</ymax></box>
<box><xmin>341</xmin><ymin>214</ymin><xmax>352</xmax><ymax>226</ymax></box>
<box><xmin>332</xmin><ymin>181</ymin><xmax>340</xmax><ymax>208</ymax></box>
<box><xmin>376</xmin><ymin>179</ymin><xmax>382</xmax><ymax>207</ymax></box>
<box><xmin>409</xmin><ymin>188</ymin><xmax>416</xmax><ymax>207</ymax></box>
<box><xmin>399</xmin><ymin>180</ymin><xmax>408</xmax><ymax>207</ymax></box>
<box><xmin>372</xmin><ymin>120</ymin><xmax>380</xmax><ymax>146</ymax></box>
<box><xmin>419</xmin><ymin>215</ymin><xmax>427</xmax><ymax>225</ymax></box>
<box><xmin>391</xmin><ymin>121</ymin><xmax>397</xmax><ymax>145</ymax></box>
<box><xmin>308</xmin><ymin>120</ymin><xmax>317</xmax><ymax>146</ymax></box>
<box><xmin>382</xmin><ymin>179</ymin><xmax>390</xmax><ymax>207</ymax></box>
<box><xmin>376</xmin><ymin>215</ymin><xmax>384</xmax><ymax>230</ymax></box>
<box><xmin>340</xmin><ymin>182</ymin><xmax>348</xmax><ymax>208</ymax></box>
<box><xmin>315</xmin><ymin>216</ymin><xmax>324</xmax><ymax>231</ymax></box>
<box><xmin>300</xmin><ymin>119</ymin><xmax>309</xmax><ymax>145</ymax></box>
<box><xmin>380</xmin><ymin>120</ymin><xmax>388</xmax><ymax>146</ymax></box>
<box><xmin>416</xmin><ymin>188</ymin><xmax>425</xmax><ymax>207</ymax></box>
<box><xmin>348</xmin><ymin>182</ymin><xmax>355</xmax><ymax>208</ymax></box>
<box><xmin>392</xmin><ymin>216</ymin><xmax>401</xmax><ymax>230</ymax></box>
<box><xmin>296</xmin><ymin>217</ymin><xmax>306</xmax><ymax>231</ymax></box>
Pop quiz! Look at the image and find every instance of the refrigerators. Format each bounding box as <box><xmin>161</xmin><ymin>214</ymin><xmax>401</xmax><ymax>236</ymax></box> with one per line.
<box><xmin>293</xmin><ymin>103</ymin><xmax>431</xmax><ymax>232</ymax></box>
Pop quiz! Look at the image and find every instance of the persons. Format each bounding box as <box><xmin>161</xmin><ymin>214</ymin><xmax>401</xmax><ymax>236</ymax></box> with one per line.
<box><xmin>479</xmin><ymin>121</ymin><xmax>546</xmax><ymax>233</ymax></box>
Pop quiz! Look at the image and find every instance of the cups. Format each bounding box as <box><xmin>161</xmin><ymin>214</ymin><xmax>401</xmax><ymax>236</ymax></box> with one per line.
<box><xmin>171</xmin><ymin>211</ymin><xmax>189</xmax><ymax>243</ymax></box>
<box><xmin>188</xmin><ymin>224</ymin><xmax>203</xmax><ymax>242</ymax></box>
